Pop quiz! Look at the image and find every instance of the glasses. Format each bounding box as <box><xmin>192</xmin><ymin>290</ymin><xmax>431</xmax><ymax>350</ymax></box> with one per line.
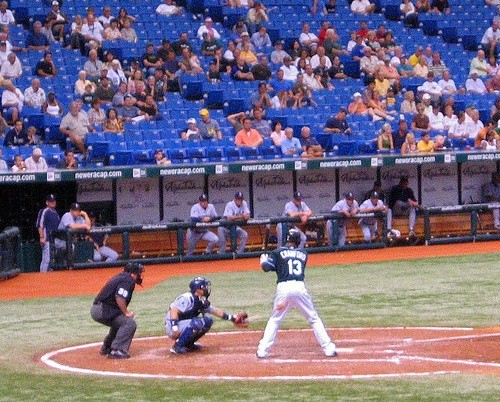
<box><xmin>417</xmin><ymin>108</ymin><xmax>424</xmax><ymax>110</ymax></box>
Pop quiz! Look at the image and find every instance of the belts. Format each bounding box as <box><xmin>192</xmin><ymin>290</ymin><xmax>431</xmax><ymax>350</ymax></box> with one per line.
<box><xmin>362</xmin><ymin>220</ymin><xmax>377</xmax><ymax>226</ymax></box>
<box><xmin>191</xmin><ymin>229</ymin><xmax>207</xmax><ymax>234</ymax></box>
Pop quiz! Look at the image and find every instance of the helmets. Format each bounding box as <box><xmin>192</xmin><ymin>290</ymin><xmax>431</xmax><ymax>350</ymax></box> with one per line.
<box><xmin>286</xmin><ymin>231</ymin><xmax>301</xmax><ymax>242</ymax></box>
<box><xmin>190</xmin><ymin>277</ymin><xmax>211</xmax><ymax>297</ymax></box>
<box><xmin>124</xmin><ymin>262</ymin><xmax>144</xmax><ymax>285</ymax></box>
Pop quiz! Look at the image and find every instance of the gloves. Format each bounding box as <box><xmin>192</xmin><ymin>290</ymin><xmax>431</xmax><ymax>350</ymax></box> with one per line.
<box><xmin>231</xmin><ymin>313</ymin><xmax>238</xmax><ymax>322</ymax></box>
<box><xmin>261</xmin><ymin>254</ymin><xmax>269</xmax><ymax>261</ymax></box>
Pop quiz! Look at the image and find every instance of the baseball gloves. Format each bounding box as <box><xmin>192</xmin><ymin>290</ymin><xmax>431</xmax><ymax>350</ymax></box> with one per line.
<box><xmin>234</xmin><ymin>313</ymin><xmax>248</xmax><ymax>329</ymax></box>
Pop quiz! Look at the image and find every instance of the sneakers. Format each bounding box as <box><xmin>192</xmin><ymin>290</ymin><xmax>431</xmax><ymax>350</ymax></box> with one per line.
<box><xmin>170</xmin><ymin>345</ymin><xmax>189</xmax><ymax>355</ymax></box>
<box><xmin>107</xmin><ymin>349</ymin><xmax>130</xmax><ymax>360</ymax></box>
<box><xmin>100</xmin><ymin>345</ymin><xmax>112</xmax><ymax>355</ymax></box>
<box><xmin>187</xmin><ymin>343</ymin><xmax>203</xmax><ymax>350</ymax></box>
<box><xmin>256</xmin><ymin>352</ymin><xmax>266</xmax><ymax>359</ymax></box>
<box><xmin>326</xmin><ymin>351</ymin><xmax>336</xmax><ymax>357</ymax></box>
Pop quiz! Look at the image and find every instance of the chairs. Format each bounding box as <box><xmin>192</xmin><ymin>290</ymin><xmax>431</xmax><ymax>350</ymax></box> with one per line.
<box><xmin>6</xmin><ymin>23</ymin><xmax>64</xmax><ymax>49</ymax></box>
<box><xmin>115</xmin><ymin>111</ymin><xmax>299</xmax><ymax>165</ymax></box>
<box><xmin>298</xmin><ymin>0</ymin><xmax>500</xmax><ymax>81</ymax></box>
<box><xmin>300</xmin><ymin>81</ymin><xmax>500</xmax><ymax>161</ymax></box>
<box><xmin>62</xmin><ymin>0</ymin><xmax>298</xmax><ymax>81</ymax></box>
<box><xmin>153</xmin><ymin>92</ymin><xmax>208</xmax><ymax>111</ymax></box>
<box><xmin>180</xmin><ymin>81</ymin><xmax>300</xmax><ymax>110</ymax></box>
<box><xmin>72</xmin><ymin>49</ymin><xmax>114</xmax><ymax>166</ymax></box>
<box><xmin>9</xmin><ymin>1</ymin><xmax>59</xmax><ymax>21</ymax></box>
<box><xmin>0</xmin><ymin>50</ymin><xmax>70</xmax><ymax>170</ymax></box>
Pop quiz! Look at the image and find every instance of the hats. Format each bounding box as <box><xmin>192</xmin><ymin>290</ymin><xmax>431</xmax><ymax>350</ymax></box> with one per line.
<box><xmin>370</xmin><ymin>190</ymin><xmax>380</xmax><ymax>198</ymax></box>
<box><xmin>293</xmin><ymin>192</ymin><xmax>304</xmax><ymax>200</ymax></box>
<box><xmin>234</xmin><ymin>192</ymin><xmax>245</xmax><ymax>200</ymax></box>
<box><xmin>146</xmin><ymin>41</ymin><xmax>152</xmax><ymax>45</ymax></box>
<box><xmin>199</xmin><ymin>193</ymin><xmax>208</xmax><ymax>201</ymax></box>
<box><xmin>352</xmin><ymin>92</ymin><xmax>361</xmax><ymax>97</ymax></box>
<box><xmin>345</xmin><ymin>192</ymin><xmax>355</xmax><ymax>200</ymax></box>
<box><xmin>401</xmin><ymin>174</ymin><xmax>408</xmax><ymax>179</ymax></box>
<box><xmin>200</xmin><ymin>108</ymin><xmax>208</xmax><ymax>116</ymax></box>
<box><xmin>203</xmin><ymin>31</ymin><xmax>205</xmax><ymax>34</ymax></box>
<box><xmin>47</xmin><ymin>91</ymin><xmax>52</xmax><ymax>93</ymax></box>
<box><xmin>33</xmin><ymin>149</ymin><xmax>44</xmax><ymax>157</ymax></box>
<box><xmin>422</xmin><ymin>92</ymin><xmax>432</xmax><ymax>100</ymax></box>
<box><xmin>374</xmin><ymin>180</ymin><xmax>378</xmax><ymax>183</ymax></box>
<box><xmin>69</xmin><ymin>202</ymin><xmax>82</xmax><ymax>210</ymax></box>
<box><xmin>163</xmin><ymin>37</ymin><xmax>169</xmax><ymax>41</ymax></box>
<box><xmin>154</xmin><ymin>149</ymin><xmax>162</xmax><ymax>154</ymax></box>
<box><xmin>241</xmin><ymin>30</ymin><xmax>248</xmax><ymax>35</ymax></box>
<box><xmin>187</xmin><ymin>117</ymin><xmax>197</xmax><ymax>124</ymax></box>
<box><xmin>43</xmin><ymin>192</ymin><xmax>58</xmax><ymax>201</ymax></box>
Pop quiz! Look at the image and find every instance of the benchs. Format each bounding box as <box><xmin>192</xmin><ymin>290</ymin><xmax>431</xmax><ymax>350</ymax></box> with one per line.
<box><xmin>347</xmin><ymin>215</ymin><xmax>426</xmax><ymax>244</ymax></box>
<box><xmin>173</xmin><ymin>224</ymin><xmax>264</xmax><ymax>253</ymax></box>
<box><xmin>430</xmin><ymin>212</ymin><xmax>499</xmax><ymax>238</ymax></box>
<box><xmin>103</xmin><ymin>229</ymin><xmax>172</xmax><ymax>257</ymax></box>
<box><xmin>267</xmin><ymin>217</ymin><xmax>348</xmax><ymax>248</ymax></box>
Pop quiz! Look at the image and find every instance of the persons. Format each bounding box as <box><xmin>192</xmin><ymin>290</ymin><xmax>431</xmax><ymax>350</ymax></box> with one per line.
<box><xmin>35</xmin><ymin>193</ymin><xmax>61</xmax><ymax>273</ymax></box>
<box><xmin>482</xmin><ymin>171</ymin><xmax>500</xmax><ymax>230</ymax></box>
<box><xmin>81</xmin><ymin>212</ymin><xmax>119</xmax><ymax>264</ymax></box>
<box><xmin>388</xmin><ymin>175</ymin><xmax>427</xmax><ymax>239</ymax></box>
<box><xmin>0</xmin><ymin>0</ymin><xmax>500</xmax><ymax>174</ymax></box>
<box><xmin>90</xmin><ymin>261</ymin><xmax>146</xmax><ymax>360</ymax></box>
<box><xmin>185</xmin><ymin>193</ymin><xmax>219</xmax><ymax>257</ymax></box>
<box><xmin>326</xmin><ymin>192</ymin><xmax>359</xmax><ymax>247</ymax></box>
<box><xmin>255</xmin><ymin>228</ymin><xmax>337</xmax><ymax>359</ymax></box>
<box><xmin>217</xmin><ymin>191</ymin><xmax>251</xmax><ymax>255</ymax></box>
<box><xmin>275</xmin><ymin>192</ymin><xmax>313</xmax><ymax>248</ymax></box>
<box><xmin>364</xmin><ymin>181</ymin><xmax>401</xmax><ymax>236</ymax></box>
<box><xmin>55</xmin><ymin>202</ymin><xmax>91</xmax><ymax>251</ymax></box>
<box><xmin>358</xmin><ymin>191</ymin><xmax>387</xmax><ymax>244</ymax></box>
<box><xmin>164</xmin><ymin>277</ymin><xmax>240</xmax><ymax>353</ymax></box>
